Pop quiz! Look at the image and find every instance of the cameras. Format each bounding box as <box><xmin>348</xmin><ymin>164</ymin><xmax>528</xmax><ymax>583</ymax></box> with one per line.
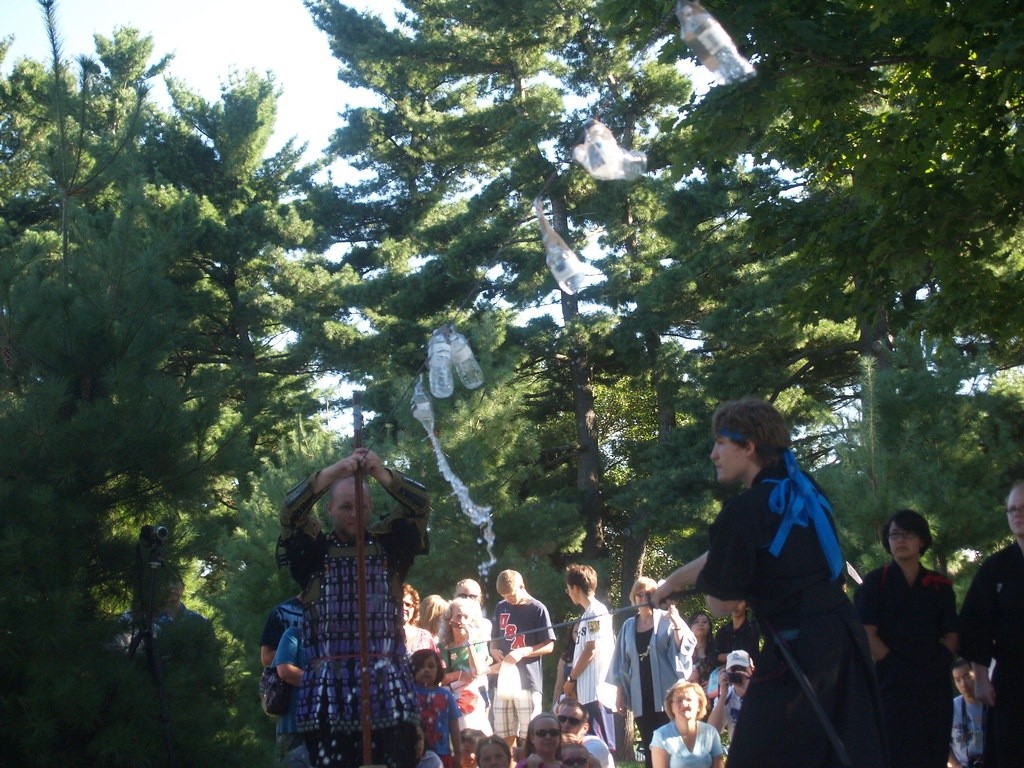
<box><xmin>726</xmin><ymin>672</ymin><xmax>742</xmax><ymax>684</ymax></box>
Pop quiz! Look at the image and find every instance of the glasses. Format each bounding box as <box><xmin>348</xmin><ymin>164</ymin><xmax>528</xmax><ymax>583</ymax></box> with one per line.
<box><xmin>1006</xmin><ymin>505</ymin><xmax>1024</xmax><ymax>513</ymax></box>
<box><xmin>636</xmin><ymin>592</ymin><xmax>650</xmax><ymax>597</ymax></box>
<box><xmin>888</xmin><ymin>528</ymin><xmax>921</xmax><ymax>539</ymax></box>
<box><xmin>557</xmin><ymin>714</ymin><xmax>585</xmax><ymax>725</ymax></box>
<box><xmin>531</xmin><ymin>729</ymin><xmax>561</xmax><ymax>737</ymax></box>
<box><xmin>454</xmin><ymin>593</ymin><xmax>480</xmax><ymax>599</ymax></box>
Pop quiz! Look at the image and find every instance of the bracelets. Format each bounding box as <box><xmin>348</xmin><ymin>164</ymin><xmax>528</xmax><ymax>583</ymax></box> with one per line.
<box><xmin>458</xmin><ymin>670</ymin><xmax>462</xmax><ymax>680</ymax></box>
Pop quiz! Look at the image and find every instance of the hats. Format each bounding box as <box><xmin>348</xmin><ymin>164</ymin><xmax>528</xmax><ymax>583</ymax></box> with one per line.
<box><xmin>726</xmin><ymin>650</ymin><xmax>749</xmax><ymax>670</ymax></box>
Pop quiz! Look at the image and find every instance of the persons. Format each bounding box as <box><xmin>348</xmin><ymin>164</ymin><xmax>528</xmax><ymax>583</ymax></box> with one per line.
<box><xmin>117</xmin><ymin>573</ymin><xmax>204</xmax><ymax>657</ymax></box>
<box><xmin>605</xmin><ymin>577</ymin><xmax>697</xmax><ymax>768</ymax></box>
<box><xmin>257</xmin><ymin>590</ymin><xmax>306</xmax><ymax>752</ymax></box>
<box><xmin>956</xmin><ymin>480</ymin><xmax>1024</xmax><ymax>768</ymax></box>
<box><xmin>460</xmin><ymin>697</ymin><xmax>614</xmax><ymax>768</ymax></box>
<box><xmin>403</xmin><ymin>579</ymin><xmax>494</xmax><ymax>738</ymax></box>
<box><xmin>649</xmin><ymin>400</ymin><xmax>892</xmax><ymax>768</ymax></box>
<box><xmin>714</xmin><ymin>599</ymin><xmax>759</xmax><ymax>657</ymax></box>
<box><xmin>853</xmin><ymin>509</ymin><xmax>958</xmax><ymax>768</ymax></box>
<box><xmin>947</xmin><ymin>658</ymin><xmax>984</xmax><ymax>768</ymax></box>
<box><xmin>414</xmin><ymin>719</ymin><xmax>444</xmax><ymax>768</ymax></box>
<box><xmin>649</xmin><ymin>682</ymin><xmax>725</xmax><ymax>768</ymax></box>
<box><xmin>706</xmin><ymin>650</ymin><xmax>755</xmax><ymax>741</ymax></box>
<box><xmin>275</xmin><ymin>447</ymin><xmax>430</xmax><ymax>768</ymax></box>
<box><xmin>490</xmin><ymin>570</ymin><xmax>557</xmax><ymax>768</ymax></box>
<box><xmin>553</xmin><ymin>564</ymin><xmax>618</xmax><ymax>752</ymax></box>
<box><xmin>409</xmin><ymin>649</ymin><xmax>462</xmax><ymax>768</ymax></box>
<box><xmin>686</xmin><ymin>610</ymin><xmax>720</xmax><ymax>722</ymax></box>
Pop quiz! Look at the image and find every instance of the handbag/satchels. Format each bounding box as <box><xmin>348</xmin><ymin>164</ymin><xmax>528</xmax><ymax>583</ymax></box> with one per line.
<box><xmin>260</xmin><ymin>667</ymin><xmax>291</xmax><ymax>716</ymax></box>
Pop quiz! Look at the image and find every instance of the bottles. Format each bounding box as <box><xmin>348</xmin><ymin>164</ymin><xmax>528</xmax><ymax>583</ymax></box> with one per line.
<box><xmin>428</xmin><ymin>329</ymin><xmax>453</xmax><ymax>398</ymax></box>
<box><xmin>410</xmin><ymin>383</ymin><xmax>431</xmax><ymax>421</ymax></box>
<box><xmin>676</xmin><ymin>4</ymin><xmax>754</xmax><ymax>89</ymax></box>
<box><xmin>538</xmin><ymin>217</ymin><xmax>587</xmax><ymax>294</ymax></box>
<box><xmin>449</xmin><ymin>334</ymin><xmax>484</xmax><ymax>390</ymax></box>
<box><xmin>587</xmin><ymin>118</ymin><xmax>622</xmax><ymax>180</ymax></box>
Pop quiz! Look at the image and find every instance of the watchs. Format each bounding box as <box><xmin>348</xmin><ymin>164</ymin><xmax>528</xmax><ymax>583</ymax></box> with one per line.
<box><xmin>567</xmin><ymin>676</ymin><xmax>575</xmax><ymax>682</ymax></box>
